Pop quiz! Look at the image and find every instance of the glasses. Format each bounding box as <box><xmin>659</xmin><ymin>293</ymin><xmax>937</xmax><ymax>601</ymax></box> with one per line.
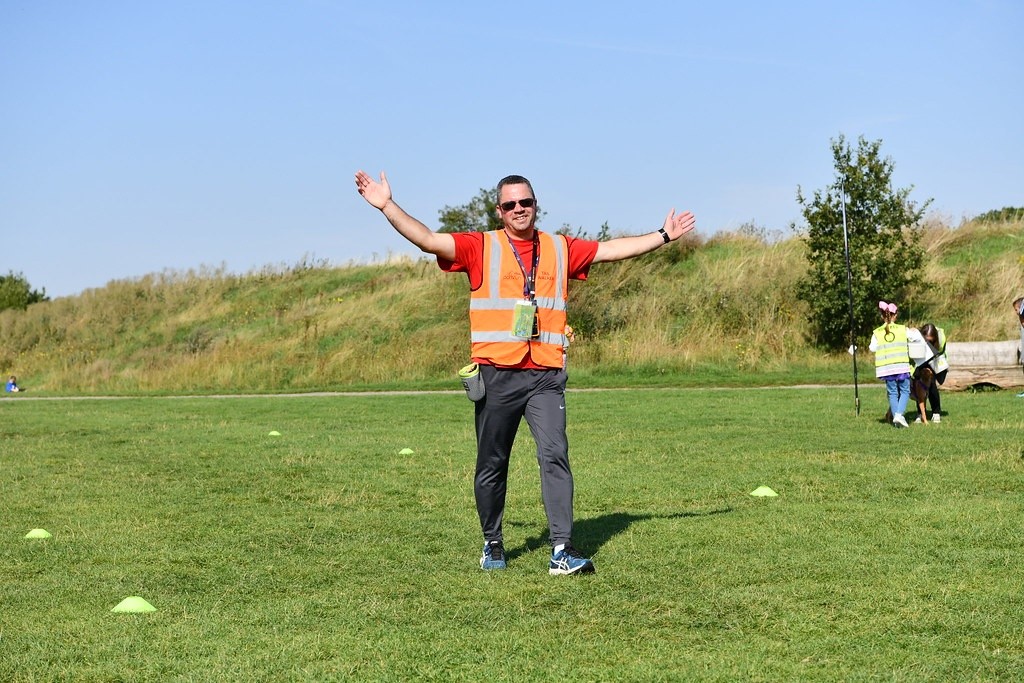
<box><xmin>499</xmin><ymin>198</ymin><xmax>537</xmax><ymax>212</ymax></box>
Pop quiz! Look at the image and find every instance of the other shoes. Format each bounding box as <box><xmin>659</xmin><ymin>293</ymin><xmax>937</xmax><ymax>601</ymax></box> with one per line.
<box><xmin>893</xmin><ymin>413</ymin><xmax>909</xmax><ymax>428</ymax></box>
<box><xmin>915</xmin><ymin>418</ymin><xmax>921</xmax><ymax>425</ymax></box>
<box><xmin>931</xmin><ymin>413</ymin><xmax>940</xmax><ymax>423</ymax></box>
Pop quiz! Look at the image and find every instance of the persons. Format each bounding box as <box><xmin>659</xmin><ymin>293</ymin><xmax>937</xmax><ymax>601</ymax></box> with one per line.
<box><xmin>915</xmin><ymin>323</ymin><xmax>949</xmax><ymax>423</ymax></box>
<box><xmin>907</xmin><ymin>328</ymin><xmax>938</xmax><ymax>426</ymax></box>
<box><xmin>355</xmin><ymin>170</ymin><xmax>695</xmax><ymax>576</ymax></box>
<box><xmin>1013</xmin><ymin>297</ymin><xmax>1024</xmax><ymax>375</ymax></box>
<box><xmin>869</xmin><ymin>301</ymin><xmax>920</xmax><ymax>429</ymax></box>
<box><xmin>5</xmin><ymin>376</ymin><xmax>21</xmax><ymax>393</ymax></box>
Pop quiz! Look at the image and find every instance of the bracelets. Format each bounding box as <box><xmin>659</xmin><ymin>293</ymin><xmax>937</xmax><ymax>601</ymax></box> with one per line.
<box><xmin>658</xmin><ymin>228</ymin><xmax>670</xmax><ymax>243</ymax></box>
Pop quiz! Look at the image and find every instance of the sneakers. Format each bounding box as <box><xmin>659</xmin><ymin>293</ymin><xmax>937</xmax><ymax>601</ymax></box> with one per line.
<box><xmin>549</xmin><ymin>542</ymin><xmax>596</xmax><ymax>576</ymax></box>
<box><xmin>479</xmin><ymin>539</ymin><xmax>507</xmax><ymax>573</ymax></box>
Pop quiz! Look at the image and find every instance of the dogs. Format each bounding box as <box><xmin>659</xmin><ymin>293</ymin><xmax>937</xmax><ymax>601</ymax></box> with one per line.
<box><xmin>886</xmin><ymin>367</ymin><xmax>934</xmax><ymax>425</ymax></box>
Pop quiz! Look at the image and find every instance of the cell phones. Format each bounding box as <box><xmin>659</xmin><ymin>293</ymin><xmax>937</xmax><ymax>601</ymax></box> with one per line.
<box><xmin>532</xmin><ymin>312</ymin><xmax>539</xmax><ymax>338</ymax></box>
